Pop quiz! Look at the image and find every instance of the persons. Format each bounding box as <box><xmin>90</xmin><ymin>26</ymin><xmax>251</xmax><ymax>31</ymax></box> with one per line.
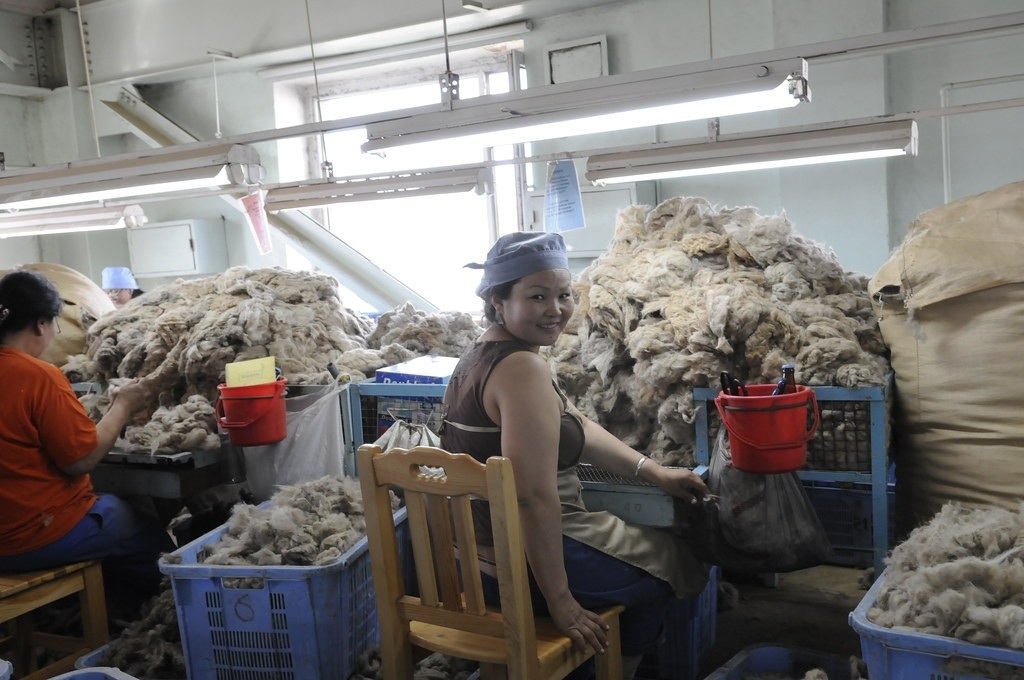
<box><xmin>438</xmin><ymin>231</ymin><xmax>712</xmax><ymax>680</ymax></box>
<box><xmin>0</xmin><ymin>270</ymin><xmax>161</xmax><ymax>620</ymax></box>
<box><xmin>101</xmin><ymin>267</ymin><xmax>145</xmax><ymax>305</ymax></box>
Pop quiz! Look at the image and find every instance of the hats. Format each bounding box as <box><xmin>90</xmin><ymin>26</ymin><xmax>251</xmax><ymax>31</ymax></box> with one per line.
<box><xmin>462</xmin><ymin>231</ymin><xmax>571</xmax><ymax>302</ymax></box>
<box><xmin>100</xmin><ymin>267</ymin><xmax>141</xmax><ymax>291</ymax></box>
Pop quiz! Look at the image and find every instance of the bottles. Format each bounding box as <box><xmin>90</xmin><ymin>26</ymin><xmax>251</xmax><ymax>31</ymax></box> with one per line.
<box><xmin>780</xmin><ymin>364</ymin><xmax>798</xmax><ymax>394</ymax></box>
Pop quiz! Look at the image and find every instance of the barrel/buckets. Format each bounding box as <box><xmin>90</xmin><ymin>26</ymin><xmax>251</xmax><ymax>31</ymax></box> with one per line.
<box><xmin>712</xmin><ymin>383</ymin><xmax>819</xmax><ymax>473</ymax></box>
<box><xmin>215</xmin><ymin>379</ymin><xmax>288</xmax><ymax>446</ymax></box>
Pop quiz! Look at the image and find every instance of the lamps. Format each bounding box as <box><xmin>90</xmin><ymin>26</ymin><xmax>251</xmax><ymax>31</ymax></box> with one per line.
<box><xmin>0</xmin><ymin>0</ymin><xmax>918</xmax><ymax>242</ymax></box>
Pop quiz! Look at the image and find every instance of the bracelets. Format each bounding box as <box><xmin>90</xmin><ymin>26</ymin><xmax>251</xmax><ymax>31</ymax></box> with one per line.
<box><xmin>634</xmin><ymin>455</ymin><xmax>648</xmax><ymax>477</ymax></box>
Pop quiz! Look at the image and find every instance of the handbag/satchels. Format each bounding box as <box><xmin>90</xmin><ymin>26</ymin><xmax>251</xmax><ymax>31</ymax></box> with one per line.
<box><xmin>242</xmin><ymin>372</ymin><xmax>352</xmax><ymax>501</ymax></box>
<box><xmin>690</xmin><ymin>420</ymin><xmax>830</xmax><ymax>574</ymax></box>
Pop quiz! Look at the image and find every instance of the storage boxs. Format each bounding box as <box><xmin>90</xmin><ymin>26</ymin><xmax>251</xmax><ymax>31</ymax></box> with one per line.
<box><xmin>376</xmin><ymin>354</ymin><xmax>461</xmax><ymax>440</ymax></box>
<box><xmin>45</xmin><ymin>495</ymin><xmax>418</xmax><ymax>680</ymax></box>
<box><xmin>571</xmin><ymin>464</ymin><xmax>1024</xmax><ymax>680</ymax></box>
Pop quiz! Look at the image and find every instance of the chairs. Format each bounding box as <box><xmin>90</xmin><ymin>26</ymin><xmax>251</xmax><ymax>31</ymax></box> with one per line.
<box><xmin>0</xmin><ymin>554</ymin><xmax>111</xmax><ymax>680</ymax></box>
<box><xmin>357</xmin><ymin>442</ymin><xmax>625</xmax><ymax>680</ymax></box>
